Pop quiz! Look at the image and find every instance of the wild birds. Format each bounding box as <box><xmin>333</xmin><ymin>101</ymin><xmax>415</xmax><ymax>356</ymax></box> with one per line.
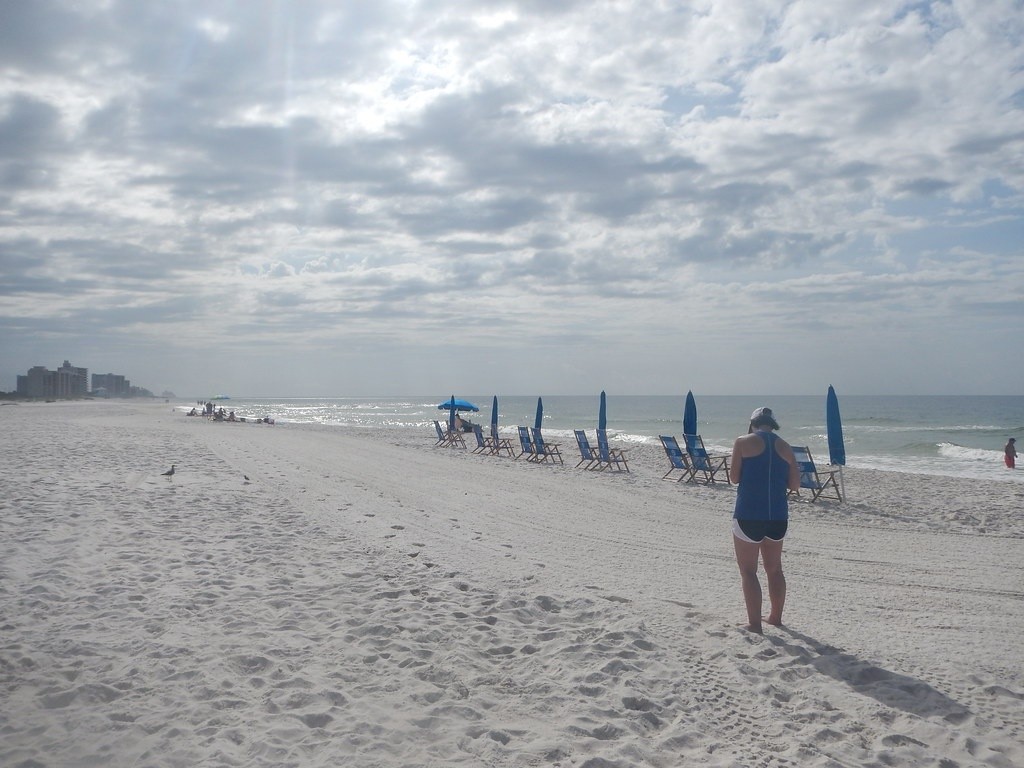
<box><xmin>161</xmin><ymin>465</ymin><xmax>177</xmax><ymax>480</ymax></box>
<box><xmin>243</xmin><ymin>475</ymin><xmax>250</xmax><ymax>481</ymax></box>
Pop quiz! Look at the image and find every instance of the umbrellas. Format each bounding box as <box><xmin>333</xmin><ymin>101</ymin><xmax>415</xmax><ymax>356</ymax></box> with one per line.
<box><xmin>491</xmin><ymin>395</ymin><xmax>498</xmax><ymax>436</ymax></box>
<box><xmin>535</xmin><ymin>397</ymin><xmax>543</xmax><ymax>429</ymax></box>
<box><xmin>683</xmin><ymin>390</ymin><xmax>697</xmax><ymax>467</ymax></box>
<box><xmin>438</xmin><ymin>399</ymin><xmax>479</xmax><ymax>414</ymax></box>
<box><xmin>599</xmin><ymin>390</ymin><xmax>606</xmax><ymax>429</ymax></box>
<box><xmin>827</xmin><ymin>385</ymin><xmax>846</xmax><ymax>503</ymax></box>
<box><xmin>450</xmin><ymin>395</ymin><xmax>455</xmax><ymax>429</ymax></box>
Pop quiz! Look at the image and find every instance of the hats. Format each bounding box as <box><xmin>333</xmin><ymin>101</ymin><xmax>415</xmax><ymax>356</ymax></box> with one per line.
<box><xmin>750</xmin><ymin>407</ymin><xmax>779</xmax><ymax>427</ymax></box>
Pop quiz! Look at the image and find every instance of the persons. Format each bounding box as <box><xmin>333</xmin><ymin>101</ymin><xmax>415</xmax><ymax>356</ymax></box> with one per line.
<box><xmin>730</xmin><ymin>408</ymin><xmax>801</xmax><ymax>634</ymax></box>
<box><xmin>190</xmin><ymin>400</ymin><xmax>235</xmax><ymax>421</ymax></box>
<box><xmin>455</xmin><ymin>414</ymin><xmax>482</xmax><ymax>428</ymax></box>
<box><xmin>1004</xmin><ymin>438</ymin><xmax>1018</xmax><ymax>468</ymax></box>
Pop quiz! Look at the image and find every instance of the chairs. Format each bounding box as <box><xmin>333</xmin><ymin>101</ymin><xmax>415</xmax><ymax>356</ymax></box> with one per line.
<box><xmin>573</xmin><ymin>430</ymin><xmax>612</xmax><ymax>470</ymax></box>
<box><xmin>445</xmin><ymin>416</ymin><xmax>480</xmax><ymax>433</ymax></box>
<box><xmin>472</xmin><ymin>423</ymin><xmax>499</xmax><ymax>455</ymax></box>
<box><xmin>487</xmin><ymin>423</ymin><xmax>516</xmax><ymax>458</ymax></box>
<box><xmin>659</xmin><ymin>435</ymin><xmax>709</xmax><ymax>484</ymax></box>
<box><xmin>589</xmin><ymin>429</ymin><xmax>630</xmax><ymax>473</ymax></box>
<box><xmin>443</xmin><ymin>420</ymin><xmax>468</xmax><ymax>449</ymax></box>
<box><xmin>433</xmin><ymin>420</ymin><xmax>458</xmax><ymax>446</ymax></box>
<box><xmin>683</xmin><ymin>434</ymin><xmax>732</xmax><ymax>486</ymax></box>
<box><xmin>788</xmin><ymin>446</ymin><xmax>842</xmax><ymax>503</ymax></box>
<box><xmin>514</xmin><ymin>426</ymin><xmax>548</xmax><ymax>462</ymax></box>
<box><xmin>529</xmin><ymin>427</ymin><xmax>563</xmax><ymax>463</ymax></box>
